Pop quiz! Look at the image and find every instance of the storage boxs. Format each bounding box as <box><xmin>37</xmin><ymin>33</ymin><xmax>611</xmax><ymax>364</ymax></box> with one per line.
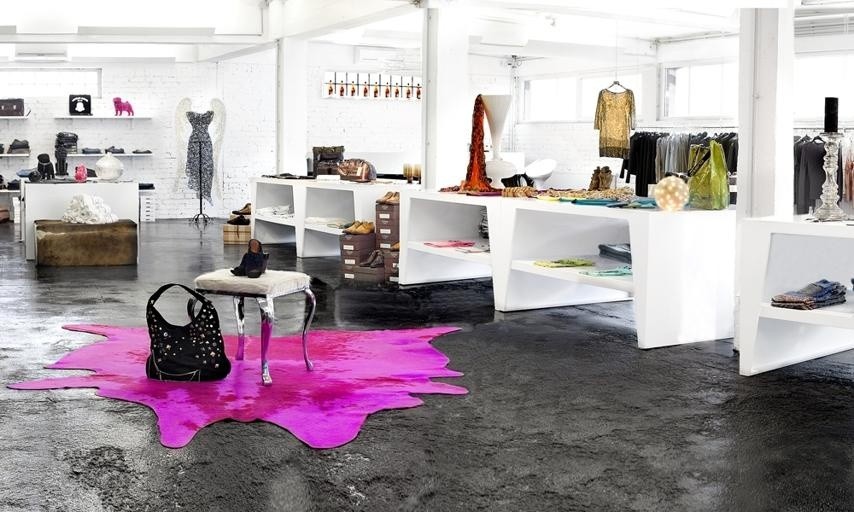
<box><xmin>376</xmin><ymin>225</ymin><xmax>399</xmax><ymax>239</ymax></box>
<box><xmin>342</xmin><ymin>266</ymin><xmax>355</xmax><ymax>279</ymax></box>
<box><xmin>224</xmin><ymin>224</ymin><xmax>251</xmax><ymax>245</ymax></box>
<box><xmin>342</xmin><ymin>251</ymin><xmax>375</xmax><ymax>266</ymax></box>
<box><xmin>339</xmin><ymin>234</ymin><xmax>375</xmax><ymax>251</ymax></box>
<box><xmin>376</xmin><ymin>205</ymin><xmax>399</xmax><ymax>224</ymax></box>
<box><xmin>354</xmin><ymin>266</ymin><xmax>384</xmax><ymax>290</ymax></box>
<box><xmin>384</xmin><ymin>274</ymin><xmax>399</xmax><ymax>287</ymax></box>
<box><xmin>376</xmin><ymin>240</ymin><xmax>399</xmax><ymax>252</ymax></box>
<box><xmin>231</xmin><ymin>215</ymin><xmax>251</xmax><ymax>220</ymax></box>
<box><xmin>384</xmin><ymin>254</ymin><xmax>399</xmax><ymax>272</ymax></box>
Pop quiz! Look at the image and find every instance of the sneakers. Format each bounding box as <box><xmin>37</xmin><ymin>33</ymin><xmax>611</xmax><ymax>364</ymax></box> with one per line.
<box><xmin>351</xmin><ymin>222</ymin><xmax>375</xmax><ymax>235</ymax></box>
<box><xmin>232</xmin><ymin>203</ymin><xmax>251</xmax><ymax>214</ymax></box>
<box><xmin>385</xmin><ymin>192</ymin><xmax>400</xmax><ymax>204</ymax></box>
<box><xmin>227</xmin><ymin>215</ymin><xmax>250</xmax><ymax>225</ymax></box>
<box><xmin>342</xmin><ymin>221</ymin><xmax>362</xmax><ymax>234</ymax></box>
<box><xmin>390</xmin><ymin>242</ymin><xmax>399</xmax><ymax>251</ymax></box>
<box><xmin>376</xmin><ymin>192</ymin><xmax>394</xmax><ymax>203</ymax></box>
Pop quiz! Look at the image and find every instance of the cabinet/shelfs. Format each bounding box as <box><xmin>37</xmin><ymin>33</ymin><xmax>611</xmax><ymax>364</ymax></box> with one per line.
<box><xmin>251</xmin><ymin>178</ymin><xmax>317</xmax><ymax>257</ymax></box>
<box><xmin>399</xmin><ymin>190</ymin><xmax>564</xmax><ymax>311</ymax></box>
<box><xmin>738</xmin><ymin>213</ymin><xmax>854</xmax><ymax>375</ymax></box>
<box><xmin>299</xmin><ymin>180</ymin><xmax>395</xmax><ymax>258</ymax></box>
<box><xmin>499</xmin><ymin>204</ymin><xmax>737</xmax><ymax>348</ymax></box>
<box><xmin>139</xmin><ymin>189</ymin><xmax>155</xmax><ymax>222</ymax></box>
<box><xmin>25</xmin><ymin>179</ymin><xmax>139</xmax><ymax>261</ymax></box>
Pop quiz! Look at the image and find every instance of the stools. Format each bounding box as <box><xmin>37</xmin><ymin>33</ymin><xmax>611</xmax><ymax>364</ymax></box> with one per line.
<box><xmin>188</xmin><ymin>267</ymin><xmax>316</xmax><ymax>386</ymax></box>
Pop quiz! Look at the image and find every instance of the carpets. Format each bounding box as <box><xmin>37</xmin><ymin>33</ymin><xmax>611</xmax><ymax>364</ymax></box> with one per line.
<box><xmin>6</xmin><ymin>324</ymin><xmax>470</xmax><ymax>449</ymax></box>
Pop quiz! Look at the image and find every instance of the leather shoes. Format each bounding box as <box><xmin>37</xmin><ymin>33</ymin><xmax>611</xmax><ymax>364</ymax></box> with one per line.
<box><xmin>360</xmin><ymin>251</ymin><xmax>378</xmax><ymax>267</ymax></box>
<box><xmin>231</xmin><ymin>253</ymin><xmax>268</xmax><ymax>276</ymax></box>
<box><xmin>246</xmin><ymin>239</ymin><xmax>264</xmax><ymax>278</ymax></box>
<box><xmin>371</xmin><ymin>253</ymin><xmax>384</xmax><ymax>268</ymax></box>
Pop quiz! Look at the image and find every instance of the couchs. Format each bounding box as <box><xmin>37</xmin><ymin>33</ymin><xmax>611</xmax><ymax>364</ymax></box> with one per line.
<box><xmin>34</xmin><ymin>218</ymin><xmax>137</xmax><ymax>267</ymax></box>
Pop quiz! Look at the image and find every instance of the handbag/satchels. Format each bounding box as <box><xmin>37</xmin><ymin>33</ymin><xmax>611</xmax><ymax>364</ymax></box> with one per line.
<box><xmin>145</xmin><ymin>283</ymin><xmax>232</xmax><ymax>382</ymax></box>
<box><xmin>688</xmin><ymin>141</ymin><xmax>730</xmax><ymax>211</ymax></box>
